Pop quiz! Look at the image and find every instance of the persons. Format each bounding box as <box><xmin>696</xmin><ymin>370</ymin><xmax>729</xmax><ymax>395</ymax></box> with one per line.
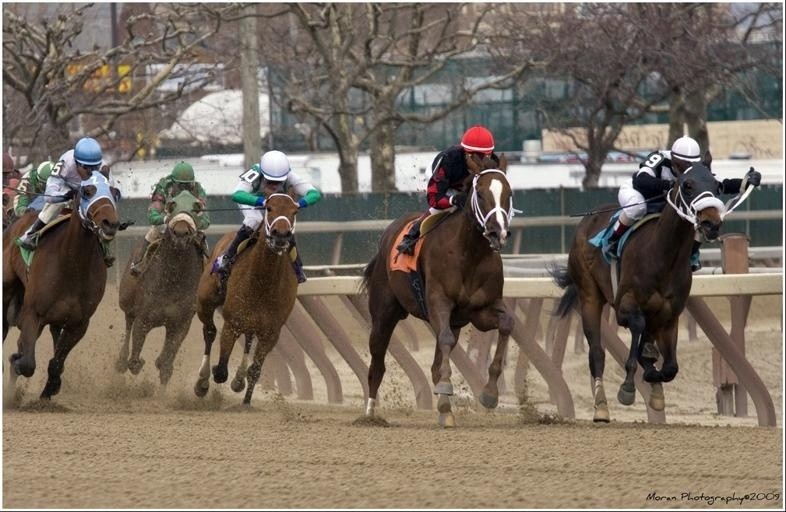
<box><xmin>14</xmin><ymin>161</ymin><xmax>56</xmax><ymax>218</ymax></box>
<box><xmin>397</xmin><ymin>123</ymin><xmax>505</xmax><ymax>254</ymax></box>
<box><xmin>3</xmin><ymin>152</ymin><xmax>19</xmax><ymax>226</ymax></box>
<box><xmin>131</xmin><ymin>160</ymin><xmax>210</xmax><ymax>269</ymax></box>
<box><xmin>215</xmin><ymin>150</ymin><xmax>322</xmax><ymax>283</ymax></box>
<box><xmin>22</xmin><ymin>137</ymin><xmax>115</xmax><ymax>262</ymax></box>
<box><xmin>604</xmin><ymin>134</ymin><xmax>700</xmax><ymax>261</ymax></box>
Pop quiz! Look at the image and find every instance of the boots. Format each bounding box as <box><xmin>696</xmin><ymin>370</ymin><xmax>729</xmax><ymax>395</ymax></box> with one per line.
<box><xmin>291</xmin><ymin>256</ymin><xmax>308</xmax><ymax>284</ymax></box>
<box><xmin>211</xmin><ymin>249</ymin><xmax>239</xmax><ymax>275</ymax></box>
<box><xmin>100</xmin><ymin>232</ymin><xmax>118</xmax><ymax>262</ymax></box>
<box><xmin>395</xmin><ymin>218</ymin><xmax>423</xmax><ymax>256</ymax></box>
<box><xmin>129</xmin><ymin>237</ymin><xmax>158</xmax><ymax>275</ymax></box>
<box><xmin>14</xmin><ymin>217</ymin><xmax>49</xmax><ymax>250</ymax></box>
<box><xmin>599</xmin><ymin>217</ymin><xmax>628</xmax><ymax>266</ymax></box>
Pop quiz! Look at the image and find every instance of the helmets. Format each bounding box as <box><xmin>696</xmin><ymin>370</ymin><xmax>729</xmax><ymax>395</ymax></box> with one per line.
<box><xmin>73</xmin><ymin>134</ymin><xmax>102</xmax><ymax>166</ymax></box>
<box><xmin>669</xmin><ymin>136</ymin><xmax>702</xmax><ymax>164</ymax></box>
<box><xmin>458</xmin><ymin>125</ymin><xmax>496</xmax><ymax>155</ymax></box>
<box><xmin>35</xmin><ymin>160</ymin><xmax>54</xmax><ymax>182</ymax></box>
<box><xmin>3</xmin><ymin>153</ymin><xmax>16</xmax><ymax>174</ymax></box>
<box><xmin>170</xmin><ymin>160</ymin><xmax>196</xmax><ymax>184</ymax></box>
<box><xmin>259</xmin><ymin>150</ymin><xmax>291</xmax><ymax>183</ymax></box>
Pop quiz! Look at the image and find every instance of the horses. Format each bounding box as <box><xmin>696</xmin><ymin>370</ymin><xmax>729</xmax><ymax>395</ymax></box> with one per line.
<box><xmin>193</xmin><ymin>183</ymin><xmax>300</xmax><ymax>408</ymax></box>
<box><xmin>3</xmin><ymin>158</ymin><xmax>121</xmax><ymax>406</ymax></box>
<box><xmin>113</xmin><ymin>178</ymin><xmax>205</xmax><ymax>387</ymax></box>
<box><xmin>355</xmin><ymin>152</ymin><xmax>516</xmax><ymax>429</ymax></box>
<box><xmin>545</xmin><ymin>148</ymin><xmax>723</xmax><ymax>425</ymax></box>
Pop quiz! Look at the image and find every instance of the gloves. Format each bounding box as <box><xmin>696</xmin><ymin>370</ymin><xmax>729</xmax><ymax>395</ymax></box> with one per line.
<box><xmin>743</xmin><ymin>166</ymin><xmax>762</xmax><ymax>189</ymax></box>
<box><xmin>448</xmin><ymin>193</ymin><xmax>469</xmax><ymax>206</ymax></box>
